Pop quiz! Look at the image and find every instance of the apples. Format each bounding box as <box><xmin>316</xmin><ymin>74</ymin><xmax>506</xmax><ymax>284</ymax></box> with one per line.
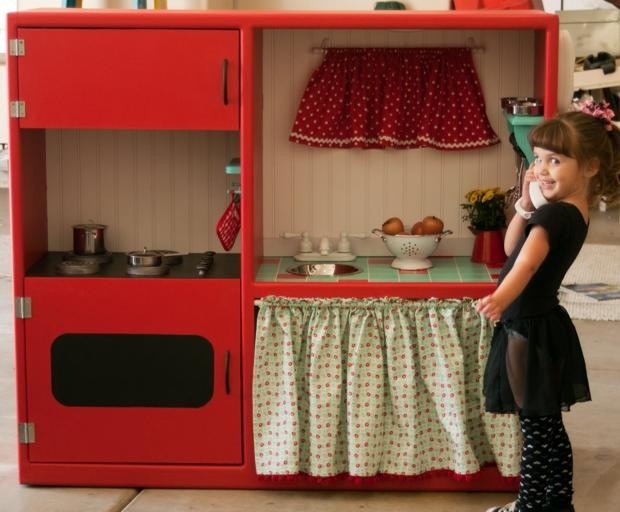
<box><xmin>382</xmin><ymin>216</ymin><xmax>443</xmax><ymax>234</ymax></box>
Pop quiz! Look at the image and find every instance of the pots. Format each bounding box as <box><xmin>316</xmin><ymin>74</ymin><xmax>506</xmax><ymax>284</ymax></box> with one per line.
<box><xmin>124</xmin><ymin>247</ymin><xmax>192</xmax><ymax>266</ymax></box>
<box><xmin>71</xmin><ymin>219</ymin><xmax>110</xmax><ymax>255</ymax></box>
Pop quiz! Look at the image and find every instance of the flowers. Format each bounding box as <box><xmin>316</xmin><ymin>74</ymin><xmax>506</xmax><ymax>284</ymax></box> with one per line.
<box><xmin>458</xmin><ymin>186</ymin><xmax>507</xmax><ymax>234</ymax></box>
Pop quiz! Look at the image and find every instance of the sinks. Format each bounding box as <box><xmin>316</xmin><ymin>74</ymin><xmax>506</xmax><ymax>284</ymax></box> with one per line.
<box><xmin>286</xmin><ymin>262</ymin><xmax>363</xmax><ymax>278</ymax></box>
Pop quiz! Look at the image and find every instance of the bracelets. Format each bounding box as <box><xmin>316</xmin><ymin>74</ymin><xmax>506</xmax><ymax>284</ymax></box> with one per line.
<box><xmin>513</xmin><ymin>195</ymin><xmax>532</xmax><ymax>220</ymax></box>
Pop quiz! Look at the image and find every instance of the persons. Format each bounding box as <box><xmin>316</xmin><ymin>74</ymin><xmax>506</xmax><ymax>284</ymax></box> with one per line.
<box><xmin>473</xmin><ymin>102</ymin><xmax>620</xmax><ymax>511</ymax></box>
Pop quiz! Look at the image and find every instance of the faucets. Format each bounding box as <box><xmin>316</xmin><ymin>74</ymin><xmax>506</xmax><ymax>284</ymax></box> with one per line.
<box><xmin>319</xmin><ymin>238</ymin><xmax>332</xmax><ymax>257</ymax></box>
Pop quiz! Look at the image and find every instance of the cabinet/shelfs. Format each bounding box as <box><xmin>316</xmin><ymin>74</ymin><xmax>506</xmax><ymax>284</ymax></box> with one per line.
<box><xmin>16</xmin><ymin>27</ymin><xmax>240</xmax><ymax>133</ymax></box>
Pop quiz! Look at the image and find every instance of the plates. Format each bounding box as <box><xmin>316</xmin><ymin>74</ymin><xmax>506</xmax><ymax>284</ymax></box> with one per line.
<box><xmin>499</xmin><ymin>96</ymin><xmax>542</xmax><ymax>116</ymax></box>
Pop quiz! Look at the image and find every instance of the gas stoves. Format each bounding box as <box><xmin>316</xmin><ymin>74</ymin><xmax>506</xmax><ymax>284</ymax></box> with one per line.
<box><xmin>27</xmin><ymin>250</ymin><xmax>243</xmax><ymax>281</ymax></box>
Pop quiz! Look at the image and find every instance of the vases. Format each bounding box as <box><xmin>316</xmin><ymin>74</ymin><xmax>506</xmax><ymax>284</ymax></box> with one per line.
<box><xmin>472</xmin><ymin>230</ymin><xmax>509</xmax><ymax>263</ymax></box>
